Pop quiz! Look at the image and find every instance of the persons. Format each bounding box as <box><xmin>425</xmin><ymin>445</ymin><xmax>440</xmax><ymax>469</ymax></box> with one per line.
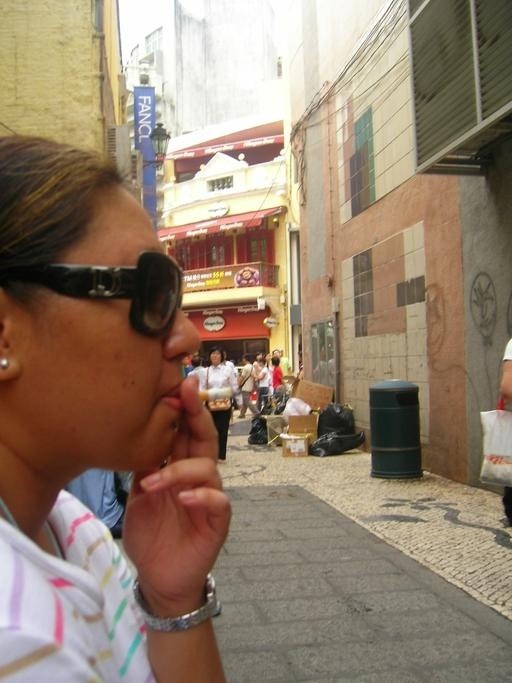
<box><xmin>0</xmin><ymin>132</ymin><xmax>234</xmax><ymax>683</ymax></box>
<box><xmin>187</xmin><ymin>356</ymin><xmax>207</xmax><ymax>391</ymax></box>
<box><xmin>500</xmin><ymin>338</ymin><xmax>512</xmax><ymax>526</ymax></box>
<box><xmin>222</xmin><ymin>349</ymin><xmax>238</xmax><ymax>378</ymax></box>
<box><xmin>184</xmin><ymin>356</ymin><xmax>193</xmax><ymax>376</ymax></box>
<box><xmin>296</xmin><ymin>351</ymin><xmax>304</xmax><ymax>379</ymax></box>
<box><xmin>199</xmin><ymin>345</ymin><xmax>243</xmax><ymax>464</ymax></box>
<box><xmin>237</xmin><ymin>349</ymin><xmax>292</xmax><ymax>418</ymax></box>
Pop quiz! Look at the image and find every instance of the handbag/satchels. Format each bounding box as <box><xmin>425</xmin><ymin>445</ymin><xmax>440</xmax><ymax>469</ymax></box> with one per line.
<box><xmin>207</xmin><ymin>399</ymin><xmax>231</xmax><ymax>412</ymax></box>
<box><xmin>479</xmin><ymin>409</ymin><xmax>512</xmax><ymax>487</ymax></box>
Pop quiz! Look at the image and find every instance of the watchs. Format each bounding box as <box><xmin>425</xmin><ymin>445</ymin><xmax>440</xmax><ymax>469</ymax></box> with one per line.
<box><xmin>131</xmin><ymin>568</ymin><xmax>223</xmax><ymax>632</ymax></box>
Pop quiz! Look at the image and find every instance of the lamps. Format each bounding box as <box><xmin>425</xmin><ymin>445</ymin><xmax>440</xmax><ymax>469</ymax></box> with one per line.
<box><xmin>143</xmin><ymin>122</ymin><xmax>171</xmax><ymax>170</ymax></box>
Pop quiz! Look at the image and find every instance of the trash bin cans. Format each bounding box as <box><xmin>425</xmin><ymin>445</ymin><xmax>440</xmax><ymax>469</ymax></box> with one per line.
<box><xmin>368</xmin><ymin>380</ymin><xmax>423</xmax><ymax>478</ymax></box>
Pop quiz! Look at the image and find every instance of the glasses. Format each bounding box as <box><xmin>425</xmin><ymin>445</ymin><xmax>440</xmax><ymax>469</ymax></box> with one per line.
<box><xmin>7</xmin><ymin>251</ymin><xmax>183</xmax><ymax>335</ymax></box>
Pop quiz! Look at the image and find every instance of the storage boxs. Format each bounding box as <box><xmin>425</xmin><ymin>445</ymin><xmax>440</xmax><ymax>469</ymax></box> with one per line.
<box><xmin>279</xmin><ymin>411</ymin><xmax>320</xmax><ymax>457</ymax></box>
